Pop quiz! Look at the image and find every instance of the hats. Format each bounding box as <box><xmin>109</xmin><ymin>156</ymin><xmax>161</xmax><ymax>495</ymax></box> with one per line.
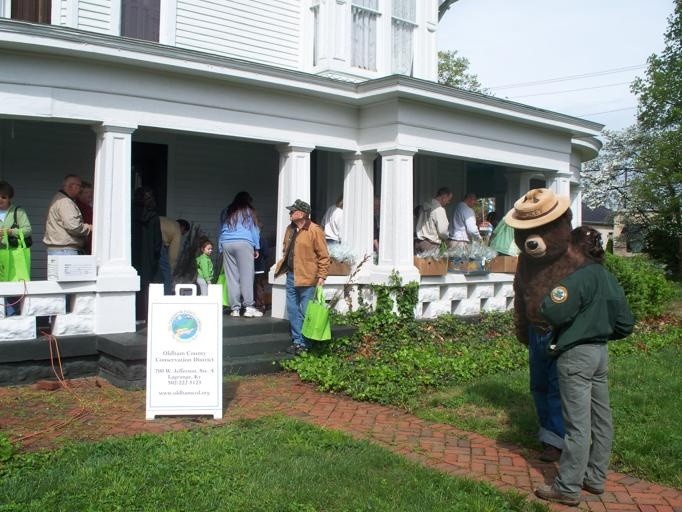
<box><xmin>285</xmin><ymin>198</ymin><xmax>311</xmax><ymax>213</ymax></box>
<box><xmin>504</xmin><ymin>187</ymin><xmax>573</xmax><ymax>232</ymax></box>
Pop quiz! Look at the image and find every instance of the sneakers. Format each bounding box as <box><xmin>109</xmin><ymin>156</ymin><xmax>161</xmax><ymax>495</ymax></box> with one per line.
<box><xmin>229</xmin><ymin>311</ymin><xmax>239</xmax><ymax>319</ymax></box>
<box><xmin>243</xmin><ymin>307</ymin><xmax>265</xmax><ymax>318</ymax></box>
<box><xmin>534</xmin><ymin>484</ymin><xmax>580</xmax><ymax>505</ymax></box>
<box><xmin>284</xmin><ymin>343</ymin><xmax>309</xmax><ymax>357</ymax></box>
<box><xmin>540</xmin><ymin>441</ymin><xmax>560</xmax><ymax>462</ymax></box>
<box><xmin>582</xmin><ymin>483</ymin><xmax>604</xmax><ymax>494</ymax></box>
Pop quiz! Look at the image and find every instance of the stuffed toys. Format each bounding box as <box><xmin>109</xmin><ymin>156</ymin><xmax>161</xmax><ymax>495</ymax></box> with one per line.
<box><xmin>503</xmin><ymin>188</ymin><xmax>578</xmax><ymax>462</ymax></box>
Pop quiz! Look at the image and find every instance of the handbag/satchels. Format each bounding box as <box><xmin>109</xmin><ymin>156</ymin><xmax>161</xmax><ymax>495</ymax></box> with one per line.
<box><xmin>7</xmin><ymin>220</ymin><xmax>34</xmax><ymax>248</ymax></box>
<box><xmin>300</xmin><ymin>299</ymin><xmax>333</xmax><ymax>342</ymax></box>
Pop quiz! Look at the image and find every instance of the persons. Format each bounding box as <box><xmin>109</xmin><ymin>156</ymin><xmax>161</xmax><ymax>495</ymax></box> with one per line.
<box><xmin>0</xmin><ymin>181</ymin><xmax>32</xmax><ymax>316</ymax></box>
<box><xmin>535</xmin><ymin>227</ymin><xmax>634</xmax><ymax>507</ymax></box>
<box><xmin>274</xmin><ymin>194</ymin><xmax>380</xmax><ymax>356</ymax></box>
<box><xmin>132</xmin><ymin>175</ymin><xmax>268</xmax><ymax>325</ymax></box>
<box><xmin>413</xmin><ymin>186</ymin><xmax>522</xmax><ymax>258</ymax></box>
<box><xmin>41</xmin><ymin>174</ymin><xmax>93</xmax><ymax>255</ymax></box>
